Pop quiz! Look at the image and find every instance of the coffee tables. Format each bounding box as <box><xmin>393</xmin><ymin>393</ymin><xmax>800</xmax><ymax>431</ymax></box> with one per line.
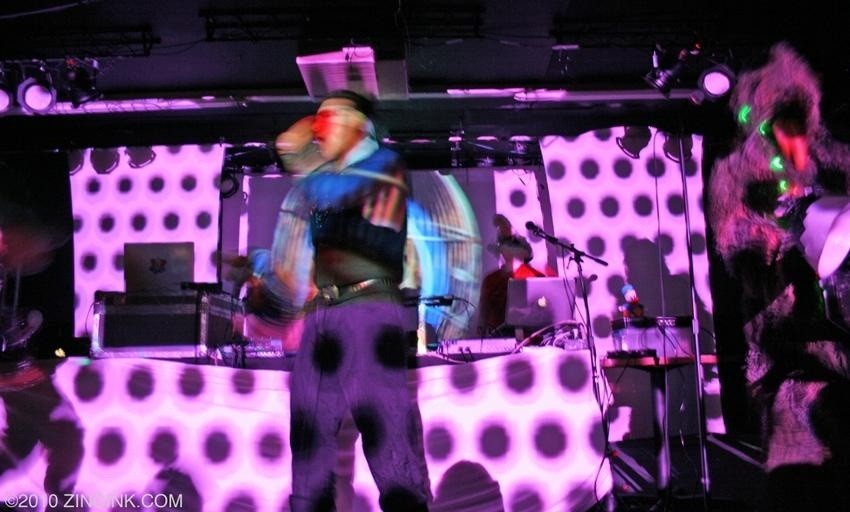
<box><xmin>1</xmin><ymin>219</ymin><xmax>53</xmax><ymax>276</ymax></box>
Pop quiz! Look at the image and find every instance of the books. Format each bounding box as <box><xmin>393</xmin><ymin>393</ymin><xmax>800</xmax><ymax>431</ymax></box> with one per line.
<box><xmin>90</xmin><ymin>289</ymin><xmax>246</xmax><ymax>365</ymax></box>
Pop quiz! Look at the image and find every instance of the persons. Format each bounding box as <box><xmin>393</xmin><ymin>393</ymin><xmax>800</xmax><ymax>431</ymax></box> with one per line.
<box><xmin>240</xmin><ymin>84</ymin><xmax>436</xmax><ymax>512</ymax></box>
<box><xmin>702</xmin><ymin>41</ymin><xmax>849</xmax><ymax>512</ymax></box>
<box><xmin>0</xmin><ymin>203</ymin><xmax>91</xmax><ymax>512</ymax></box>
<box><xmin>141</xmin><ymin>463</ymin><xmax>204</xmax><ymax>512</ymax></box>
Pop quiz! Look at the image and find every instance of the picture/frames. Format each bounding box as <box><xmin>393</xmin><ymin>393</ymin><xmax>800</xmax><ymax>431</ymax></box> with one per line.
<box><xmin>597</xmin><ymin>354</ymin><xmax>719</xmax><ymax>511</ymax></box>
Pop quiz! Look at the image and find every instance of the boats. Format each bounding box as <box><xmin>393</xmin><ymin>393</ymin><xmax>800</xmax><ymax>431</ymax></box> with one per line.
<box><xmin>604</xmin><ymin>366</ymin><xmax>653</xmax><ymax>443</ymax></box>
<box><xmin>89</xmin><ymin>288</ymin><xmax>245</xmax><ymax>364</ymax></box>
<box><xmin>664</xmin><ymin>365</ymin><xmax>698</xmax><ymax>439</ymax></box>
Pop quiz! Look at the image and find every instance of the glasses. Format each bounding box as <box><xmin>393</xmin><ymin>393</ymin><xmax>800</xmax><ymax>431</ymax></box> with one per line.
<box><xmin>641</xmin><ymin>32</ymin><xmax>737</xmax><ymax>99</ymax></box>
<box><xmin>0</xmin><ymin>59</ymin><xmax>104</xmax><ymax>115</ymax></box>
<box><xmin>740</xmin><ymin>179</ymin><xmax>850</xmax><ymax>281</ymax></box>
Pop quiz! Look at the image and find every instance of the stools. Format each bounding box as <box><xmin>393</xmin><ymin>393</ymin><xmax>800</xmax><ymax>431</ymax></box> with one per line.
<box><xmin>319</xmin><ymin>277</ymin><xmax>386</xmax><ymax>306</ymax></box>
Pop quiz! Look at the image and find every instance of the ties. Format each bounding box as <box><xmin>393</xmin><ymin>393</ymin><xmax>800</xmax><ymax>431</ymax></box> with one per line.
<box><xmin>123</xmin><ymin>241</ymin><xmax>196</xmax><ymax>292</ymax></box>
<box><xmin>505</xmin><ymin>276</ymin><xmax>576</xmax><ymax>326</ymax></box>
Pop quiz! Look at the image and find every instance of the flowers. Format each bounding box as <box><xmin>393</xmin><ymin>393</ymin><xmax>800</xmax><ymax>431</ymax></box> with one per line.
<box><xmin>524</xmin><ymin>221</ymin><xmax>544</xmax><ymax>233</ymax></box>
